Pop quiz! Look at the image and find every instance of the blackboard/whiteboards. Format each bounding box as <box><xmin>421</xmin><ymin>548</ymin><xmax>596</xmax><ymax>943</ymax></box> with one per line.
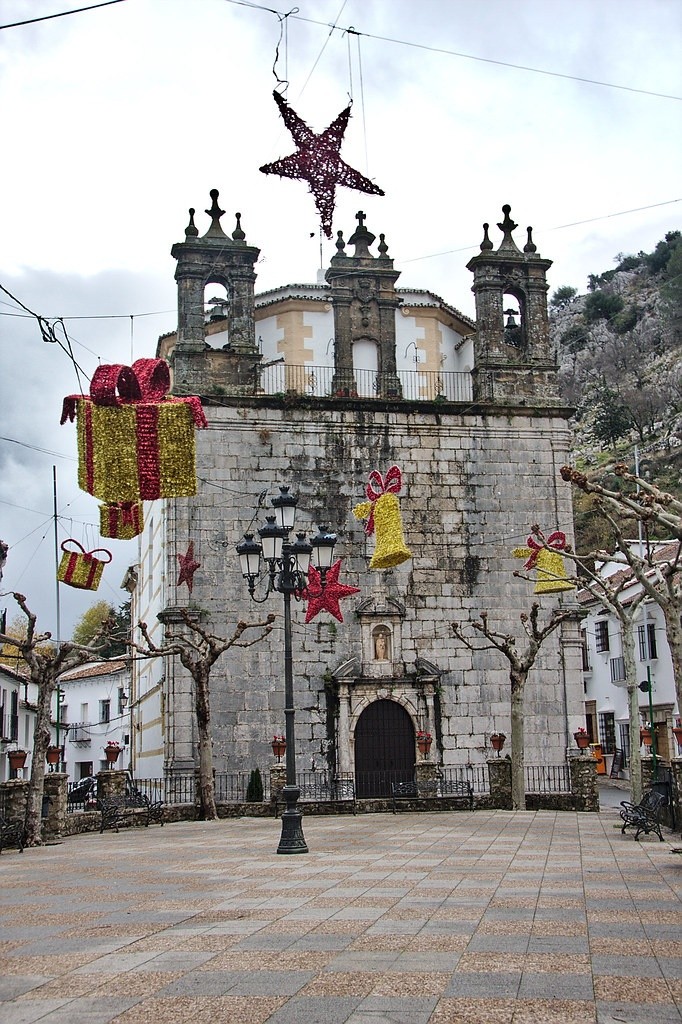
<box><xmin>610</xmin><ymin>747</ymin><xmax>623</xmax><ymax>779</ymax></box>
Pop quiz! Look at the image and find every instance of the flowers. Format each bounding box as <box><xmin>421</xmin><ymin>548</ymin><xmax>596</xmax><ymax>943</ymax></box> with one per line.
<box><xmin>4</xmin><ymin>742</ymin><xmax>32</xmax><ymax>754</ymax></box>
<box><xmin>273</xmin><ymin>734</ymin><xmax>286</xmax><ymax>741</ymax></box>
<box><xmin>577</xmin><ymin>726</ymin><xmax>590</xmax><ymax>735</ymax></box>
<box><xmin>489</xmin><ymin>727</ymin><xmax>507</xmax><ymax>736</ymax></box>
<box><xmin>106</xmin><ymin>739</ymin><xmax>122</xmax><ymax>747</ymax></box>
<box><xmin>416</xmin><ymin>730</ymin><xmax>433</xmax><ymax>739</ymax></box>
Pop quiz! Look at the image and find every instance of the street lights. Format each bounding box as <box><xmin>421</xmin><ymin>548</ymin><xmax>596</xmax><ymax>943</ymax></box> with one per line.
<box><xmin>236</xmin><ymin>485</ymin><xmax>339</xmax><ymax>854</ymax></box>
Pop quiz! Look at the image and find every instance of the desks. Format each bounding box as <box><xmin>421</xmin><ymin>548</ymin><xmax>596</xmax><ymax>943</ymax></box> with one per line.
<box><xmin>66</xmin><ymin>783</ymin><xmax>91</xmax><ymax>812</ymax></box>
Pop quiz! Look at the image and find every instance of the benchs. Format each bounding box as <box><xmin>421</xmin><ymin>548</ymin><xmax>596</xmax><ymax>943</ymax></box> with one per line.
<box><xmin>618</xmin><ymin>789</ymin><xmax>665</xmax><ymax>842</ymax></box>
<box><xmin>100</xmin><ymin>794</ymin><xmax>166</xmax><ymax>832</ymax></box>
<box><xmin>391</xmin><ymin>779</ymin><xmax>476</xmax><ymax>816</ymax></box>
<box><xmin>0</xmin><ymin>819</ymin><xmax>26</xmax><ymax>854</ymax></box>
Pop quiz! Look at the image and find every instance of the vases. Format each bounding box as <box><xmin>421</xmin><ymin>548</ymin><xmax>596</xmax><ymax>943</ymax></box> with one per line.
<box><xmin>416</xmin><ymin>736</ymin><xmax>432</xmax><ymax>754</ymax></box>
<box><xmin>104</xmin><ymin>746</ymin><xmax>119</xmax><ymax>761</ymax></box>
<box><xmin>271</xmin><ymin>741</ymin><xmax>287</xmax><ymax>756</ymax></box>
<box><xmin>491</xmin><ymin>734</ymin><xmax>506</xmax><ymax>750</ymax></box>
<box><xmin>9</xmin><ymin>752</ymin><xmax>27</xmax><ymax>770</ymax></box>
<box><xmin>575</xmin><ymin>735</ymin><xmax>591</xmax><ymax>749</ymax></box>
<box><xmin>46</xmin><ymin>749</ymin><xmax>62</xmax><ymax>763</ymax></box>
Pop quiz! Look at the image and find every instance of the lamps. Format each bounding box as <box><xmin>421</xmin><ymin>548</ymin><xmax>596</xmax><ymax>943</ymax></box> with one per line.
<box><xmin>119</xmin><ymin>564</ymin><xmax>143</xmax><ymax>595</ymax></box>
<box><xmin>117</xmin><ymin>692</ymin><xmax>132</xmax><ymax>711</ymax></box>
<box><xmin>332</xmin><ymin>656</ymin><xmax>360</xmax><ymax>677</ymax></box>
<box><xmin>416</xmin><ymin>657</ymin><xmax>444</xmax><ymax>675</ymax></box>
